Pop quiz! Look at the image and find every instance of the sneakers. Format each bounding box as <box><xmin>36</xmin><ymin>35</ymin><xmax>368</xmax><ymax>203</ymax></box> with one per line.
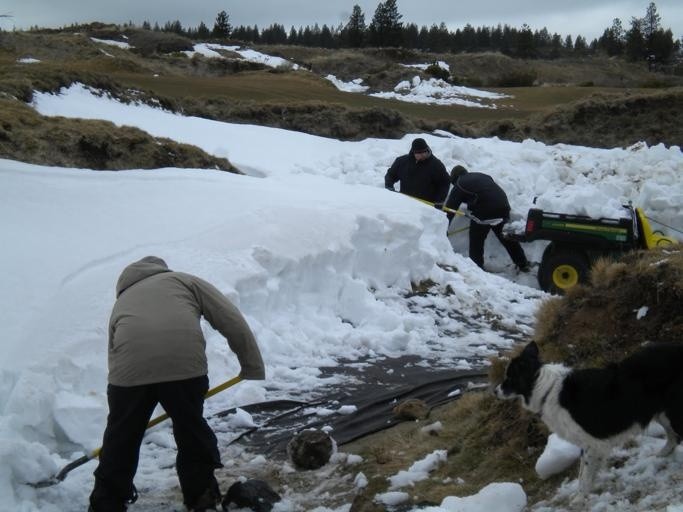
<box><xmin>515</xmin><ymin>257</ymin><xmax>529</xmax><ymax>271</ymax></box>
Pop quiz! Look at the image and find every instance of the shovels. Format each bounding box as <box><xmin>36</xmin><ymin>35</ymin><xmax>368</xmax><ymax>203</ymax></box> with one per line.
<box><xmin>397</xmin><ymin>191</ymin><xmax>504</xmax><ymax>226</ymax></box>
<box><xmin>26</xmin><ymin>378</ymin><xmax>242</xmax><ymax>488</ymax></box>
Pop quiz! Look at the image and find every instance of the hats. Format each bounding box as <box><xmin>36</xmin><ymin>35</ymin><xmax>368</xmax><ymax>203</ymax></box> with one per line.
<box><xmin>411</xmin><ymin>138</ymin><xmax>427</xmax><ymax>153</ymax></box>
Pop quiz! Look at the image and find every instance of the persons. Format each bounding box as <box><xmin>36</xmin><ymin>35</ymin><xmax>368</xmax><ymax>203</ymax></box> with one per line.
<box><xmin>81</xmin><ymin>255</ymin><xmax>268</xmax><ymax>512</ymax></box>
<box><xmin>441</xmin><ymin>164</ymin><xmax>531</xmax><ymax>273</ymax></box>
<box><xmin>385</xmin><ymin>137</ymin><xmax>451</xmax><ymax>210</ymax></box>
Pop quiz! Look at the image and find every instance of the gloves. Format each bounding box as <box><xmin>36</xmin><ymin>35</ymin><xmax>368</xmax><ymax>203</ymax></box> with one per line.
<box><xmin>434</xmin><ymin>199</ymin><xmax>442</xmax><ymax>209</ymax></box>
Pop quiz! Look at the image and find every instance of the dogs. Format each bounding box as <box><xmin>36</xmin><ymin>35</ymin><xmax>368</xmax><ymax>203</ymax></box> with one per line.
<box><xmin>493</xmin><ymin>339</ymin><xmax>683</xmax><ymax>512</ymax></box>
